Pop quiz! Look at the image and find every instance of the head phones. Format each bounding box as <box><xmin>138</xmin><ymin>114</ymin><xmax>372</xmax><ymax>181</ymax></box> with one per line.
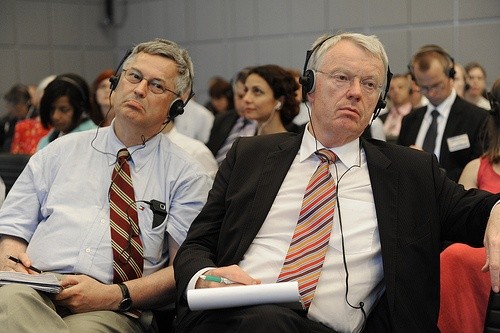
<box><xmin>109</xmin><ymin>46</ymin><xmax>194</xmax><ymax>121</ymax></box>
<box><xmin>407</xmin><ymin>48</ymin><xmax>456</xmax><ymax>81</ymax></box>
<box><xmin>298</xmin><ymin>34</ymin><xmax>393</xmax><ymax>117</ymax></box>
<box><xmin>57</xmin><ymin>76</ymin><xmax>88</xmax><ymax>112</ymax></box>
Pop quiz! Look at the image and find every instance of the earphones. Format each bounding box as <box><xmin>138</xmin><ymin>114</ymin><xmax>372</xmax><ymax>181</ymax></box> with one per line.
<box><xmin>275</xmin><ymin>101</ymin><xmax>282</xmax><ymax>109</ymax></box>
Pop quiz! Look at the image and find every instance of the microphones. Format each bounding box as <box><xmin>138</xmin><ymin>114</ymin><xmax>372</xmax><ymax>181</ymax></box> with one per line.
<box><xmin>163</xmin><ymin>114</ymin><xmax>178</xmax><ymax>125</ymax></box>
<box><xmin>373</xmin><ymin>107</ymin><xmax>382</xmax><ymax>121</ymax></box>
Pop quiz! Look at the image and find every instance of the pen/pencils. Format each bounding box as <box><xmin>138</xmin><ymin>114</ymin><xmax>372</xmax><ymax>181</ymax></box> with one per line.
<box><xmin>6</xmin><ymin>255</ymin><xmax>45</xmax><ymax>273</ymax></box>
<box><xmin>199</xmin><ymin>275</ymin><xmax>236</xmax><ymax>284</ymax></box>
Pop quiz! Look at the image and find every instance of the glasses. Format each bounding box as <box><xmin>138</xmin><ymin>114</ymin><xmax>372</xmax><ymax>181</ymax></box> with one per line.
<box><xmin>315</xmin><ymin>70</ymin><xmax>382</xmax><ymax>96</ymax></box>
<box><xmin>121</xmin><ymin>67</ymin><xmax>179</xmax><ymax>97</ymax></box>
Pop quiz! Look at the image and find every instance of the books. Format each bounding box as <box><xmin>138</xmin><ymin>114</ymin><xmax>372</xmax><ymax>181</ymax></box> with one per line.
<box><xmin>0</xmin><ymin>271</ymin><xmax>64</xmax><ymax>294</ymax></box>
<box><xmin>187</xmin><ymin>281</ymin><xmax>306</xmax><ymax>311</ymax></box>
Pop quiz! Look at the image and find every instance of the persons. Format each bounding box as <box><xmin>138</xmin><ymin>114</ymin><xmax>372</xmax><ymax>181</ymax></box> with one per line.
<box><xmin>397</xmin><ymin>45</ymin><xmax>490</xmax><ymax>183</ymax></box>
<box><xmin>175</xmin><ymin>65</ymin><xmax>313</xmax><ymax>163</ymax></box>
<box><xmin>453</xmin><ymin>65</ymin><xmax>492</xmax><ymax>111</ymax></box>
<box><xmin>0</xmin><ymin>39</ymin><xmax>218</xmax><ymax>333</ymax></box>
<box><xmin>0</xmin><ymin>71</ymin><xmax>114</xmax><ymax>154</ymax></box>
<box><xmin>173</xmin><ymin>33</ymin><xmax>500</xmax><ymax>333</ymax></box>
<box><xmin>437</xmin><ymin>78</ymin><xmax>500</xmax><ymax>333</ymax></box>
<box><xmin>369</xmin><ymin>76</ymin><xmax>428</xmax><ymax>143</ymax></box>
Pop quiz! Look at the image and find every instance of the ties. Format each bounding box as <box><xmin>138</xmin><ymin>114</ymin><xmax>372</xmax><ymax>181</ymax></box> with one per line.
<box><xmin>422</xmin><ymin>110</ymin><xmax>439</xmax><ymax>154</ymax></box>
<box><xmin>278</xmin><ymin>148</ymin><xmax>340</xmax><ymax>317</ymax></box>
<box><xmin>386</xmin><ymin>113</ymin><xmax>399</xmax><ymax>141</ymax></box>
<box><xmin>109</xmin><ymin>149</ymin><xmax>145</xmax><ymax>320</ymax></box>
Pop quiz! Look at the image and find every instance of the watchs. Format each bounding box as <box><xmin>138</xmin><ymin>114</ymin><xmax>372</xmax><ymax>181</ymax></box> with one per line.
<box><xmin>118</xmin><ymin>282</ymin><xmax>133</xmax><ymax>312</ymax></box>
